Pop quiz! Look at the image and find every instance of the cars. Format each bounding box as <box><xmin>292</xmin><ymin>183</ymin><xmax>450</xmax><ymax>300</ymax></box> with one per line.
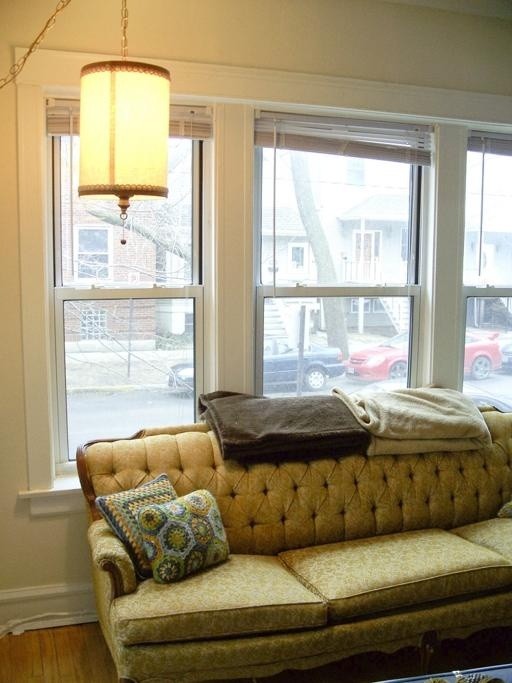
<box><xmin>168</xmin><ymin>337</ymin><xmax>346</xmax><ymax>393</ymax></box>
<box><xmin>346</xmin><ymin>328</ymin><xmax>512</xmax><ymax>383</ymax></box>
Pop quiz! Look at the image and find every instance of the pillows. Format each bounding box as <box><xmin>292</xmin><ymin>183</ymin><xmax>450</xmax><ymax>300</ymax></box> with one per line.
<box><xmin>94</xmin><ymin>472</ymin><xmax>229</xmax><ymax>585</ymax></box>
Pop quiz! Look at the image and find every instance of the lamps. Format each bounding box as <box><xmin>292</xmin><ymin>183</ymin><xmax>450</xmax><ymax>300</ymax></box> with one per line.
<box><xmin>1</xmin><ymin>0</ymin><xmax>171</xmax><ymax>245</ymax></box>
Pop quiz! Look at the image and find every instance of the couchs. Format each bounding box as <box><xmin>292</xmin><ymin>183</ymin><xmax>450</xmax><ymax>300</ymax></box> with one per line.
<box><xmin>75</xmin><ymin>384</ymin><xmax>510</xmax><ymax>680</ymax></box>
